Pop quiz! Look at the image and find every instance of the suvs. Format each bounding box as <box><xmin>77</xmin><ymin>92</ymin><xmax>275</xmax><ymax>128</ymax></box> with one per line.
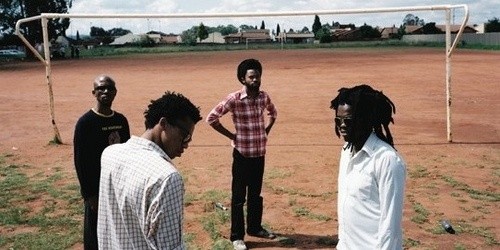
<box><xmin>12</xmin><ymin>50</ymin><xmax>26</xmax><ymax>61</ymax></box>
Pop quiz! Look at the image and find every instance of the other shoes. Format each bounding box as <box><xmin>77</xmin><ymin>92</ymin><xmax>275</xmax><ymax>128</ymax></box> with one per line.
<box><xmin>249</xmin><ymin>229</ymin><xmax>275</xmax><ymax>239</ymax></box>
<box><xmin>233</xmin><ymin>239</ymin><xmax>247</xmax><ymax>250</ymax></box>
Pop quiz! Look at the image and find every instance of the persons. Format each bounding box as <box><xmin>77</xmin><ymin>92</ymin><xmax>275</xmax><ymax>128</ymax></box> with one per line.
<box><xmin>329</xmin><ymin>84</ymin><xmax>407</xmax><ymax>250</ymax></box>
<box><xmin>59</xmin><ymin>44</ymin><xmax>80</xmax><ymax>60</ymax></box>
<box><xmin>205</xmin><ymin>58</ymin><xmax>278</xmax><ymax>250</ymax></box>
<box><xmin>74</xmin><ymin>76</ymin><xmax>130</xmax><ymax>250</ymax></box>
<box><xmin>96</xmin><ymin>90</ymin><xmax>203</xmax><ymax>250</ymax></box>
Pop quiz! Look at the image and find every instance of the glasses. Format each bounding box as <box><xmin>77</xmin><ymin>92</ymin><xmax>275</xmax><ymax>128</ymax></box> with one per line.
<box><xmin>334</xmin><ymin>117</ymin><xmax>357</xmax><ymax>126</ymax></box>
<box><xmin>95</xmin><ymin>87</ymin><xmax>115</xmax><ymax>92</ymax></box>
<box><xmin>176</xmin><ymin>124</ymin><xmax>193</xmax><ymax>143</ymax></box>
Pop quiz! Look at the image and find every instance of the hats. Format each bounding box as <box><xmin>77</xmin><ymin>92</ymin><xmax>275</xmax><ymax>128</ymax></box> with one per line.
<box><xmin>237</xmin><ymin>58</ymin><xmax>262</xmax><ymax>84</ymax></box>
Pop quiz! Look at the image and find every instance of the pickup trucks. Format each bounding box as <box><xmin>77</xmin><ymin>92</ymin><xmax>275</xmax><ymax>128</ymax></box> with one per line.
<box><xmin>0</xmin><ymin>50</ymin><xmax>18</xmax><ymax>61</ymax></box>
